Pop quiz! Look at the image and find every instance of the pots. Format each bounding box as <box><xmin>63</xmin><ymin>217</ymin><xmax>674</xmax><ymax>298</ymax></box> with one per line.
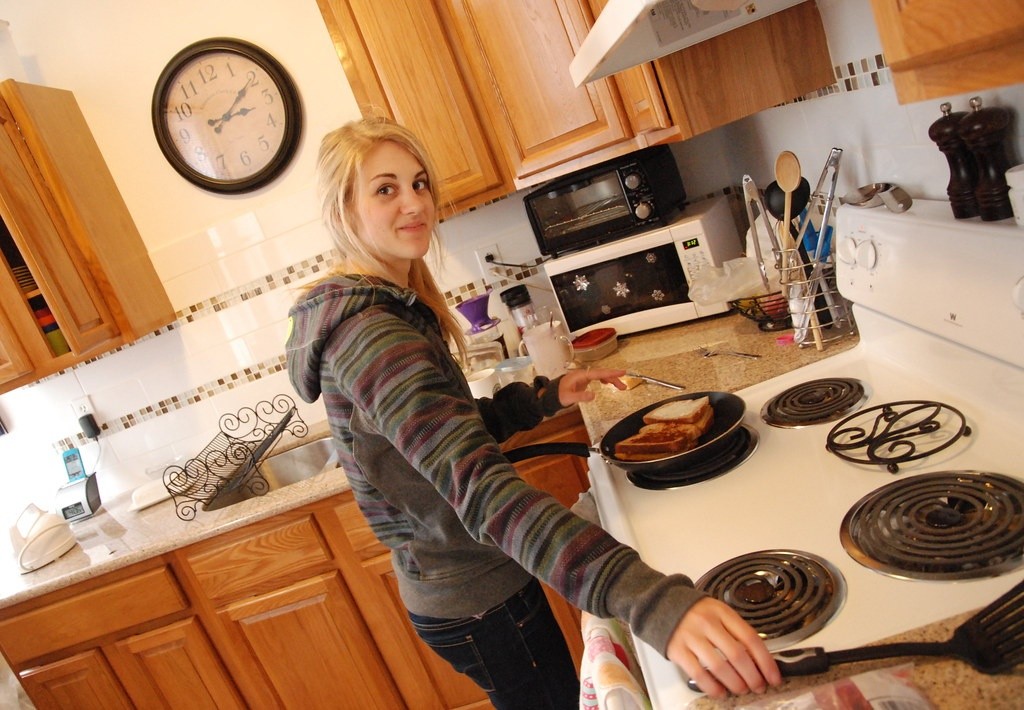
<box><xmin>502</xmin><ymin>391</ymin><xmax>747</xmax><ymax>482</ymax></box>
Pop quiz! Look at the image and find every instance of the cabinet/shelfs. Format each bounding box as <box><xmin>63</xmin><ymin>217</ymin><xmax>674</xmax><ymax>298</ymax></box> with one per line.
<box><xmin>184</xmin><ymin>492</ymin><xmax>484</xmax><ymax>709</ymax></box>
<box><xmin>0</xmin><ymin>566</ymin><xmax>270</xmax><ymax>710</ymax></box>
<box><xmin>0</xmin><ymin>77</ymin><xmax>177</xmax><ymax>395</ymax></box>
<box><xmin>589</xmin><ymin>1</ymin><xmax>838</xmax><ymax>147</ymax></box>
<box><xmin>497</xmin><ymin>406</ymin><xmax>596</xmax><ymax>678</ymax></box>
<box><xmin>317</xmin><ymin>0</ymin><xmax>632</xmax><ymax>221</ymax></box>
<box><xmin>869</xmin><ymin>0</ymin><xmax>1024</xmax><ymax>106</ymax></box>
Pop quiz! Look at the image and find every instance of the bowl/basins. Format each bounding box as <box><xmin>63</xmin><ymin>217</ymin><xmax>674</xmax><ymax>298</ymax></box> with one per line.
<box><xmin>571</xmin><ymin>328</ymin><xmax>618</xmax><ymax>361</ymax></box>
<box><xmin>495</xmin><ymin>356</ymin><xmax>534</xmax><ymax>388</ymax></box>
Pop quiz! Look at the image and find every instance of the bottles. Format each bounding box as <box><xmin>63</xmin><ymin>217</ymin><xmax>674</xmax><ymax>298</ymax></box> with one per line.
<box><xmin>1006</xmin><ymin>163</ymin><xmax>1024</xmax><ymax>226</ymax></box>
<box><xmin>34</xmin><ymin>308</ymin><xmax>69</xmax><ymax>357</ymax></box>
<box><xmin>499</xmin><ymin>284</ymin><xmax>538</xmax><ymax>340</ymax></box>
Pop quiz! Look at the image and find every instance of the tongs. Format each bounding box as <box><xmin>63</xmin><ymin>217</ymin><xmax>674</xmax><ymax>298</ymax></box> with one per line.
<box><xmin>790</xmin><ymin>148</ymin><xmax>843</xmax><ymax>296</ymax></box>
<box><xmin>742</xmin><ymin>173</ymin><xmax>782</xmax><ymax>293</ymax></box>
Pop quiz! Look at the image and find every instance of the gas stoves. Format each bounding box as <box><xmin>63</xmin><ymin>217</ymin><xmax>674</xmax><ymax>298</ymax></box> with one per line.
<box><xmin>582</xmin><ymin>195</ymin><xmax>1023</xmax><ymax>710</ymax></box>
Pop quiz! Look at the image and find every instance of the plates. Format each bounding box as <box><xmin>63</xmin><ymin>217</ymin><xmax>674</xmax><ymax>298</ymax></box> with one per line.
<box><xmin>11</xmin><ymin>265</ymin><xmax>38</xmax><ymax>293</ymax></box>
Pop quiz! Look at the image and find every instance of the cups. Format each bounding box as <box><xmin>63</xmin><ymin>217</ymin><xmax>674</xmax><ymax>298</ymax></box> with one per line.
<box><xmin>455</xmin><ymin>294</ymin><xmax>494</xmax><ymax>331</ymax></box>
<box><xmin>466</xmin><ymin>369</ymin><xmax>499</xmax><ymax>399</ymax></box>
<box><xmin>522</xmin><ymin>321</ymin><xmax>575</xmax><ymax>381</ymax></box>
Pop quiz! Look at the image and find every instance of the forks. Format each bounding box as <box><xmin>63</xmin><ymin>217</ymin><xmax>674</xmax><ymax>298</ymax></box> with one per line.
<box><xmin>692</xmin><ymin>346</ymin><xmax>761</xmax><ymax>359</ymax></box>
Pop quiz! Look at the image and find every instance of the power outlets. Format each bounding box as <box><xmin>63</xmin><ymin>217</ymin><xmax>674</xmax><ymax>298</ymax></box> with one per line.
<box><xmin>71</xmin><ymin>395</ymin><xmax>101</xmax><ymax>427</ymax></box>
<box><xmin>473</xmin><ymin>243</ymin><xmax>507</xmax><ymax>288</ymax></box>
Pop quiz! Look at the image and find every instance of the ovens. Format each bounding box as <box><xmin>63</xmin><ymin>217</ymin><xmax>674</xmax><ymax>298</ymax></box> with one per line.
<box><xmin>522</xmin><ymin>143</ymin><xmax>688</xmax><ymax>255</ymax></box>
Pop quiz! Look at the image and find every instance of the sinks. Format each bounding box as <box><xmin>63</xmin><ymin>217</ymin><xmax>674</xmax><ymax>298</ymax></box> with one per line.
<box><xmin>201</xmin><ymin>433</ymin><xmax>337</xmax><ymax>512</ymax></box>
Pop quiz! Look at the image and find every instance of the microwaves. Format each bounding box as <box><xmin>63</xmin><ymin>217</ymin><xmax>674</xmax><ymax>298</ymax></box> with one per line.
<box><xmin>543</xmin><ymin>196</ymin><xmax>745</xmax><ymax>338</ymax></box>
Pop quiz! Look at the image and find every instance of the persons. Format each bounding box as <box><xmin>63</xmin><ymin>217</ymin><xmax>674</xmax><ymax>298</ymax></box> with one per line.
<box><xmin>286</xmin><ymin>118</ymin><xmax>780</xmax><ymax>710</ymax></box>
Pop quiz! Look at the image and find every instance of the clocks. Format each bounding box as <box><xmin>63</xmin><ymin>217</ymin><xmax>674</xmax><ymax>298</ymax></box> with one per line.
<box><xmin>153</xmin><ymin>38</ymin><xmax>302</xmax><ymax>195</ymax></box>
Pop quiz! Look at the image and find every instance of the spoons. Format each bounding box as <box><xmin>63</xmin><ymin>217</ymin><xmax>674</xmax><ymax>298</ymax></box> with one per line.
<box><xmin>774</xmin><ymin>150</ymin><xmax>801</xmax><ymax>295</ymax></box>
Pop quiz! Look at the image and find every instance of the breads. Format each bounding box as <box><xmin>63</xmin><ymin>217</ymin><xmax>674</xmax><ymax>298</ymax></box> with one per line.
<box><xmin>614</xmin><ymin>396</ymin><xmax>714</xmax><ymax>461</ymax></box>
